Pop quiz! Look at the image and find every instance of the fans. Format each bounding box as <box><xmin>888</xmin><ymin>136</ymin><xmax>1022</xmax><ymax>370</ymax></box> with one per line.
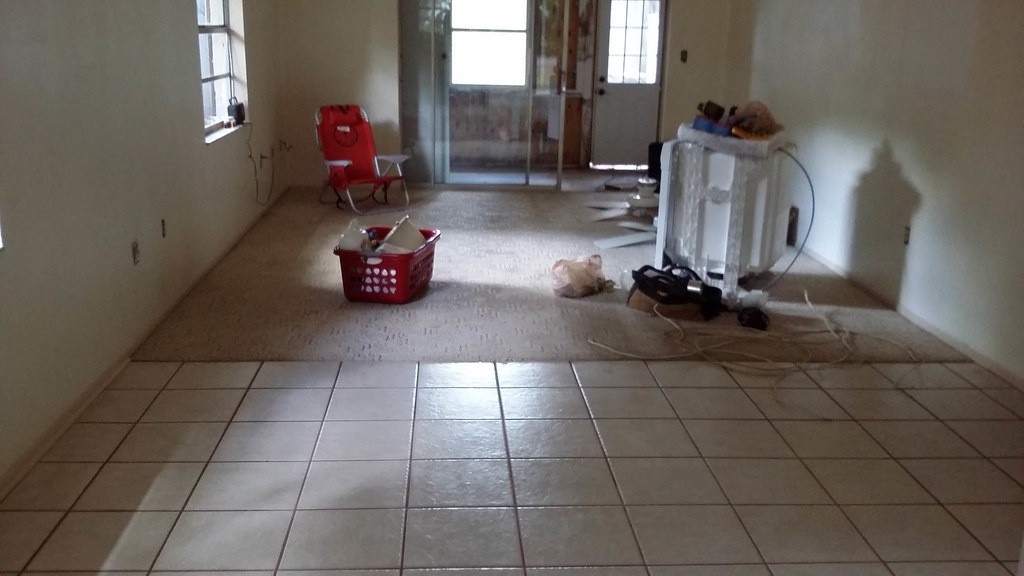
<box><xmin>585</xmin><ymin>177</ymin><xmax>659</xmax><ymax>222</ymax></box>
<box><xmin>594</xmin><ymin>214</ymin><xmax>658</xmax><ymax>250</ymax></box>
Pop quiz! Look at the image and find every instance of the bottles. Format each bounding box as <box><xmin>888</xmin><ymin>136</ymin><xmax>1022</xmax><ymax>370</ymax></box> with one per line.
<box><xmin>339</xmin><ymin>220</ymin><xmax>368</xmax><ymax>253</ymax></box>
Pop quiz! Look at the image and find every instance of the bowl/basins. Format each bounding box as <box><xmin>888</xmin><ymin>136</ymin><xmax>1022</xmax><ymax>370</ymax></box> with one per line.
<box><xmin>383</xmin><ymin>216</ymin><xmax>425</xmax><ymax>250</ymax></box>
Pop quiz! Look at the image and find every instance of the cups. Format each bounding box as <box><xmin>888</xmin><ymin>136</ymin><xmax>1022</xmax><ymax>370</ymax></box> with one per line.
<box><xmin>638</xmin><ymin>178</ymin><xmax>657</xmax><ymax>197</ymax></box>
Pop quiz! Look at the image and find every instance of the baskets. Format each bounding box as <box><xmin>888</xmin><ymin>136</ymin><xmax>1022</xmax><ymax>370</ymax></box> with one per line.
<box><xmin>333</xmin><ymin>225</ymin><xmax>442</xmax><ymax>305</ymax></box>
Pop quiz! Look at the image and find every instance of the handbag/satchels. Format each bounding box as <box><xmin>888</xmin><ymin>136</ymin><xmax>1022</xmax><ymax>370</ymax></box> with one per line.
<box><xmin>550</xmin><ymin>254</ymin><xmax>605</xmax><ymax>298</ymax></box>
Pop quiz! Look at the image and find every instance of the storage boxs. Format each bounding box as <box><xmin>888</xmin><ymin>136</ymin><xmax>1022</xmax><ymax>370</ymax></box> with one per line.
<box><xmin>333</xmin><ymin>226</ymin><xmax>442</xmax><ymax>304</ymax></box>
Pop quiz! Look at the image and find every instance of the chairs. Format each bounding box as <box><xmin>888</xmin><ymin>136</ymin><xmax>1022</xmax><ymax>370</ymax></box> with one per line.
<box><xmin>318</xmin><ymin>103</ymin><xmax>411</xmax><ymax>213</ymax></box>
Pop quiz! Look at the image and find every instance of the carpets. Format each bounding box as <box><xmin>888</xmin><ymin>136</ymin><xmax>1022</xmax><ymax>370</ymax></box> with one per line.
<box><xmin>131</xmin><ymin>175</ymin><xmax>977</xmax><ymax>366</ymax></box>
